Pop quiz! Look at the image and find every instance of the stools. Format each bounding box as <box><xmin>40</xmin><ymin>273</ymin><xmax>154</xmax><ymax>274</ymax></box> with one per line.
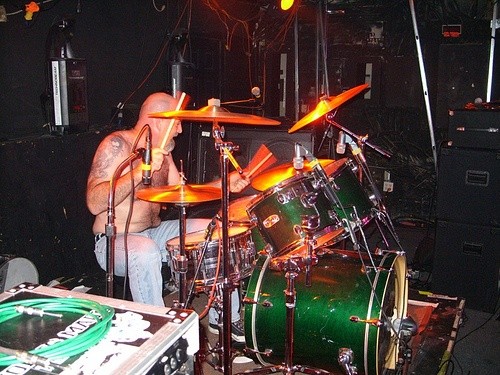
<box><xmin>105</xmin><ymin>266</ymin><xmax>171</xmax><ymax>299</ymax></box>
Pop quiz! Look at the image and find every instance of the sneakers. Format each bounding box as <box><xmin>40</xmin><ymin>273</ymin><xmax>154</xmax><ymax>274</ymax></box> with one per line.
<box><xmin>207</xmin><ymin>319</ymin><xmax>246</xmax><ymax>342</ymax></box>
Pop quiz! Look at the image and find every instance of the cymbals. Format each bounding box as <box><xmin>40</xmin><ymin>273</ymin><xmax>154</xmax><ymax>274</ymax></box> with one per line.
<box><xmin>250</xmin><ymin>158</ymin><xmax>335</xmax><ymax>193</ymax></box>
<box><xmin>143</xmin><ymin>105</ymin><xmax>282</xmax><ymax>127</ymax></box>
<box><xmin>135</xmin><ymin>183</ymin><xmax>223</xmax><ymax>205</ymax></box>
<box><xmin>286</xmin><ymin>82</ymin><xmax>370</xmax><ymax>134</ymax></box>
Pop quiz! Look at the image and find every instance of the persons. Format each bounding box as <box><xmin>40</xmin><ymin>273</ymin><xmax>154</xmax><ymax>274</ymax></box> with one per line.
<box><xmin>86</xmin><ymin>92</ymin><xmax>251</xmax><ymax>308</ymax></box>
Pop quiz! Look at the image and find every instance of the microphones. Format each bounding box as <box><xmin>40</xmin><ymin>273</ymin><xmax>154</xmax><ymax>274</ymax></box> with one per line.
<box><xmin>293</xmin><ymin>142</ymin><xmax>305</xmax><ymax>169</ymax></box>
<box><xmin>142</xmin><ymin>126</ymin><xmax>152</xmax><ymax>185</ymax></box>
<box><xmin>252</xmin><ymin>86</ymin><xmax>263</xmax><ymax>106</ymax></box>
<box><xmin>205</xmin><ymin>220</ymin><xmax>215</xmax><ymax>241</ymax></box>
<box><xmin>335</xmin><ymin>128</ymin><xmax>347</xmax><ymax>154</ymax></box>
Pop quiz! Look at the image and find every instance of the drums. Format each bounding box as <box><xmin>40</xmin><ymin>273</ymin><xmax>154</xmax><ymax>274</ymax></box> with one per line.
<box><xmin>244</xmin><ymin>171</ymin><xmax>346</xmax><ymax>262</ymax></box>
<box><xmin>315</xmin><ymin>157</ymin><xmax>378</xmax><ymax>249</ymax></box>
<box><xmin>241</xmin><ymin>248</ymin><xmax>410</xmax><ymax>375</ymax></box>
<box><xmin>164</xmin><ymin>225</ymin><xmax>254</xmax><ymax>293</ymax></box>
<box><xmin>218</xmin><ymin>193</ymin><xmax>272</xmax><ymax>253</ymax></box>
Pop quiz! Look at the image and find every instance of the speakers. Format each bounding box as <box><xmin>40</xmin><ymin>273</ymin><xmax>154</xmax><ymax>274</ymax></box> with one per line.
<box><xmin>194</xmin><ymin>124</ymin><xmax>314</xmax><ymax>198</ymax></box>
<box><xmin>429</xmin><ymin>147</ymin><xmax>500</xmax><ymax>313</ymax></box>
<box><xmin>347</xmin><ymin>224</ymin><xmax>434</xmax><ymax>283</ymax></box>
